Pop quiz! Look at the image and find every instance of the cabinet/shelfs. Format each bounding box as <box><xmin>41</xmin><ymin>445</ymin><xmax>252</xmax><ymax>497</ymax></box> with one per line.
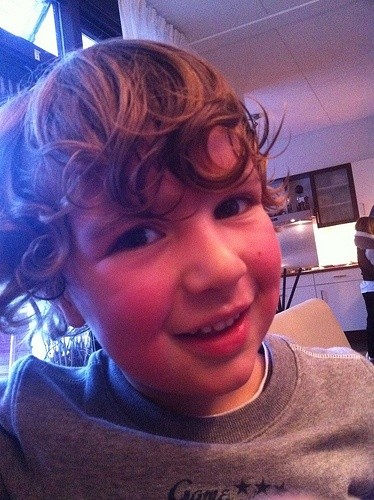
<box><xmin>262</xmin><ymin>162</ymin><xmax>373</xmax><ymax>338</ymax></box>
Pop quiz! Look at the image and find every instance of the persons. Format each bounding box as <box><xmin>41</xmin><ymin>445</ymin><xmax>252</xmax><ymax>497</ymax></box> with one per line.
<box><xmin>354</xmin><ymin>218</ymin><xmax>374</xmax><ymax>361</ymax></box>
<box><xmin>0</xmin><ymin>37</ymin><xmax>374</xmax><ymax>499</ymax></box>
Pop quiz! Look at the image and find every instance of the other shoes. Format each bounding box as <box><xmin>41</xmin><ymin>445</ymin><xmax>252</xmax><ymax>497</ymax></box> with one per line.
<box><xmin>365</xmin><ymin>352</ymin><xmax>373</xmax><ymax>361</ymax></box>
